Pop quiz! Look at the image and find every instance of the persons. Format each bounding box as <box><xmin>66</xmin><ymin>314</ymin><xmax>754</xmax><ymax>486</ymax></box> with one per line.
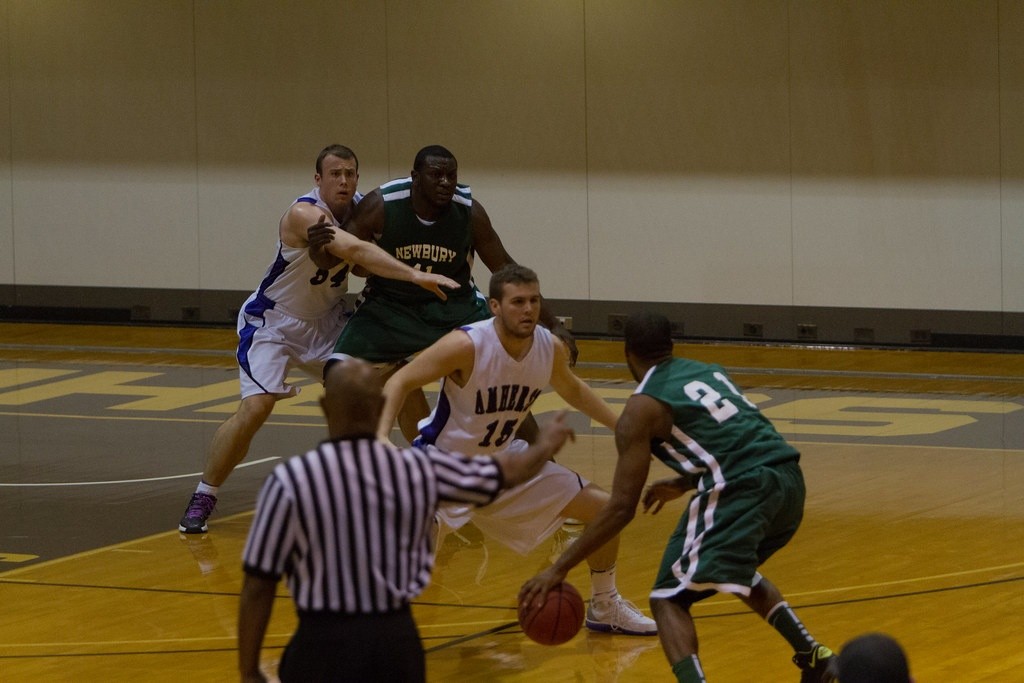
<box><xmin>834</xmin><ymin>633</ymin><xmax>916</xmax><ymax>683</ymax></box>
<box><xmin>376</xmin><ymin>265</ymin><xmax>661</xmax><ymax>636</ymax></box>
<box><xmin>180</xmin><ymin>145</ymin><xmax>461</xmax><ymax>533</ymax></box>
<box><xmin>237</xmin><ymin>356</ymin><xmax>575</xmax><ymax>683</ymax></box>
<box><xmin>306</xmin><ymin>147</ymin><xmax>580</xmax><ymax>468</ymax></box>
<box><xmin>519</xmin><ymin>313</ymin><xmax>839</xmax><ymax>683</ymax></box>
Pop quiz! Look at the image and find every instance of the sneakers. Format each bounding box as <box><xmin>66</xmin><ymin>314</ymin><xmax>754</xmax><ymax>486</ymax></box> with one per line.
<box><xmin>792</xmin><ymin>645</ymin><xmax>839</xmax><ymax>683</ymax></box>
<box><xmin>179</xmin><ymin>492</ymin><xmax>217</xmax><ymax>534</ymax></box>
<box><xmin>585</xmin><ymin>594</ymin><xmax>659</xmax><ymax>636</ymax></box>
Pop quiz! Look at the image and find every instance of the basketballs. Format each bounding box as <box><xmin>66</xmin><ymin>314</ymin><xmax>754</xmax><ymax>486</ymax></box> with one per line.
<box><xmin>517</xmin><ymin>581</ymin><xmax>585</xmax><ymax>645</ymax></box>
